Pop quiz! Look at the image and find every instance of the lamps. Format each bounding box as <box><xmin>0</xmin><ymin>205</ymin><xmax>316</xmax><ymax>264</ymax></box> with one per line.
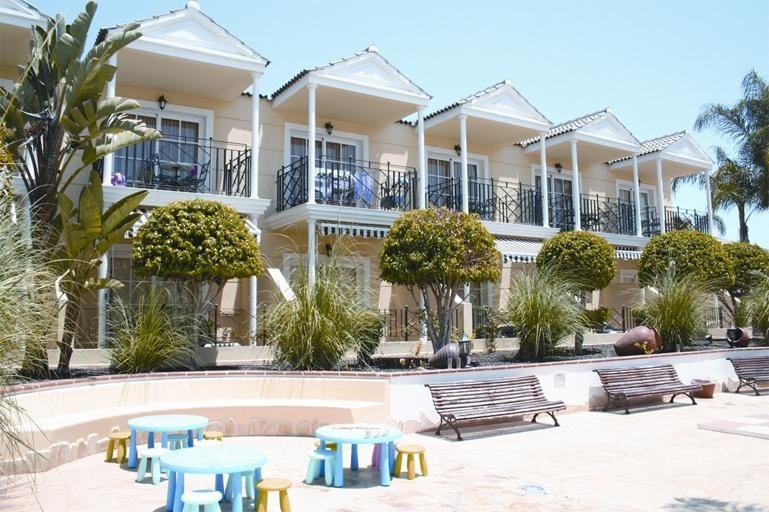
<box><xmin>555</xmin><ymin>161</ymin><xmax>563</xmax><ymax>173</ymax></box>
<box><xmin>158</xmin><ymin>94</ymin><xmax>168</xmax><ymax>109</ymax></box>
<box><xmin>326</xmin><ymin>120</ymin><xmax>334</xmax><ymax>135</ymax></box>
<box><xmin>325</xmin><ymin>243</ymin><xmax>333</xmax><ymax>257</ymax></box>
<box><xmin>639</xmin><ymin>178</ymin><xmax>642</xmax><ymax>186</ymax></box>
<box><xmin>454</xmin><ymin>142</ymin><xmax>462</xmax><ymax>156</ymax></box>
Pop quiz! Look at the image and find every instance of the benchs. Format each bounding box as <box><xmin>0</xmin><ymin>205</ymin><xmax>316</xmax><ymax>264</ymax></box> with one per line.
<box><xmin>423</xmin><ymin>374</ymin><xmax>567</xmax><ymax>440</ymax></box>
<box><xmin>592</xmin><ymin>363</ymin><xmax>703</xmax><ymax>414</ymax></box>
<box><xmin>726</xmin><ymin>356</ymin><xmax>769</xmax><ymax>395</ymax></box>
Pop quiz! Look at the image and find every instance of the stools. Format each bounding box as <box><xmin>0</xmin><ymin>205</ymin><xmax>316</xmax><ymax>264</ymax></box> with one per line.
<box><xmin>306</xmin><ymin>448</ymin><xmax>337</xmax><ymax>485</ymax></box>
<box><xmin>315</xmin><ymin>441</ymin><xmax>337</xmax><ymax>450</ymax></box>
<box><xmin>394</xmin><ymin>445</ymin><xmax>428</xmax><ymax>480</ymax></box>
<box><xmin>371</xmin><ymin>441</ymin><xmax>398</xmax><ymax>469</ymax></box>
<box><xmin>105</xmin><ymin>415</ymin><xmax>292</xmax><ymax>512</ymax></box>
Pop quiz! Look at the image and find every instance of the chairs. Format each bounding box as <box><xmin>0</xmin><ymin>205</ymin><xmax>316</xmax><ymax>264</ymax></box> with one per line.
<box><xmin>144</xmin><ymin>153</ymin><xmax>211</xmax><ymax>191</ymax></box>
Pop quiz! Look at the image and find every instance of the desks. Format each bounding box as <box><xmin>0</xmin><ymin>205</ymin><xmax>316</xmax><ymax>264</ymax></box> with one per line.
<box><xmin>315</xmin><ymin>422</ymin><xmax>401</xmax><ymax>486</ymax></box>
